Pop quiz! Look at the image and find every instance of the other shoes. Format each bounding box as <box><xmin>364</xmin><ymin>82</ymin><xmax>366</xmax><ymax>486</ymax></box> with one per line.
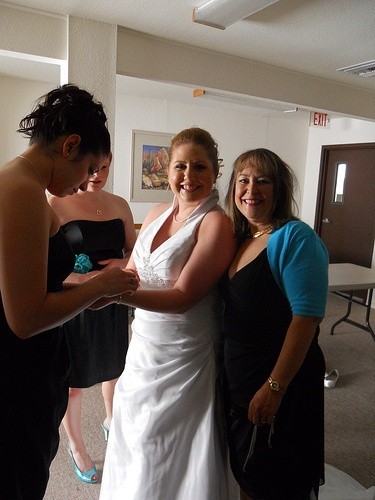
<box><xmin>324</xmin><ymin>368</ymin><xmax>339</xmax><ymax>388</ymax></box>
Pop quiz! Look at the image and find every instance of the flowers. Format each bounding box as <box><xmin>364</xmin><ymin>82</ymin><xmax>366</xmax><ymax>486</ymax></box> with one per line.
<box><xmin>73</xmin><ymin>253</ymin><xmax>93</xmax><ymax>274</ymax></box>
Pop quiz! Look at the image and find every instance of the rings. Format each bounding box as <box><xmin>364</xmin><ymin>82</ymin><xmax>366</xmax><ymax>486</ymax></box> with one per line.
<box><xmin>130</xmin><ymin>271</ymin><xmax>133</xmax><ymax>277</ymax></box>
<box><xmin>131</xmin><ymin>277</ymin><xmax>135</xmax><ymax>283</ymax></box>
<box><xmin>261</xmin><ymin>416</ymin><xmax>265</xmax><ymax>420</ymax></box>
<box><xmin>127</xmin><ymin>283</ymin><xmax>130</xmax><ymax>290</ymax></box>
<box><xmin>261</xmin><ymin>421</ymin><xmax>265</xmax><ymax>424</ymax></box>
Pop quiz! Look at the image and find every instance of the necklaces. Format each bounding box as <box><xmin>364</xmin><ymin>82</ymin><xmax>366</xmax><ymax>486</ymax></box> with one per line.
<box><xmin>251</xmin><ymin>224</ymin><xmax>274</xmax><ymax>239</ymax></box>
<box><xmin>17</xmin><ymin>155</ymin><xmax>47</xmax><ymax>191</ymax></box>
<box><xmin>173</xmin><ymin>206</ymin><xmax>188</xmax><ymax>223</ymax></box>
<box><xmin>96</xmin><ymin>206</ymin><xmax>107</xmax><ymax>215</ymax></box>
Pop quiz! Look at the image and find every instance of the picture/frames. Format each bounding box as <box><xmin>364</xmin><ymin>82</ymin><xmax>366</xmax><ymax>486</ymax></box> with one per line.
<box><xmin>129</xmin><ymin>129</ymin><xmax>176</xmax><ymax>204</ymax></box>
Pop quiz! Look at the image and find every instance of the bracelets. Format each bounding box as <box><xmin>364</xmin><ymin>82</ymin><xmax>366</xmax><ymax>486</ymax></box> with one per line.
<box><xmin>117</xmin><ymin>295</ymin><xmax>121</xmax><ymax>304</ymax></box>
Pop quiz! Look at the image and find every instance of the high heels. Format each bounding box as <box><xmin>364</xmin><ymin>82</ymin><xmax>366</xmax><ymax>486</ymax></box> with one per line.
<box><xmin>101</xmin><ymin>418</ymin><xmax>113</xmax><ymax>439</ymax></box>
<box><xmin>66</xmin><ymin>441</ymin><xmax>100</xmax><ymax>483</ymax></box>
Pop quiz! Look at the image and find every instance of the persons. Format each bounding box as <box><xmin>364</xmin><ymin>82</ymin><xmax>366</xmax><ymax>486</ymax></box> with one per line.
<box><xmin>86</xmin><ymin>127</ymin><xmax>238</xmax><ymax>500</ymax></box>
<box><xmin>48</xmin><ymin>150</ymin><xmax>136</xmax><ymax>484</ymax></box>
<box><xmin>0</xmin><ymin>82</ymin><xmax>141</xmax><ymax>500</ymax></box>
<box><xmin>218</xmin><ymin>148</ymin><xmax>329</xmax><ymax>500</ymax></box>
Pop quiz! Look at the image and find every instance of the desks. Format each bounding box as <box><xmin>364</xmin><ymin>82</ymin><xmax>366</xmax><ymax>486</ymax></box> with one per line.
<box><xmin>318</xmin><ymin>263</ymin><xmax>375</xmax><ymax>343</ymax></box>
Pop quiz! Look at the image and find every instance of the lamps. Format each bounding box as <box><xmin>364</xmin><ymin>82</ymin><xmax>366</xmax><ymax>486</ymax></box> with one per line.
<box><xmin>193</xmin><ymin>89</ymin><xmax>298</xmax><ymax>113</ymax></box>
<box><xmin>193</xmin><ymin>0</ymin><xmax>280</xmax><ymax>30</ymax></box>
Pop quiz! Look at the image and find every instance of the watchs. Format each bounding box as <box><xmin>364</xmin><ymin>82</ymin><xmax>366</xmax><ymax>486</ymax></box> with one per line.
<box><xmin>268</xmin><ymin>377</ymin><xmax>285</xmax><ymax>396</ymax></box>
